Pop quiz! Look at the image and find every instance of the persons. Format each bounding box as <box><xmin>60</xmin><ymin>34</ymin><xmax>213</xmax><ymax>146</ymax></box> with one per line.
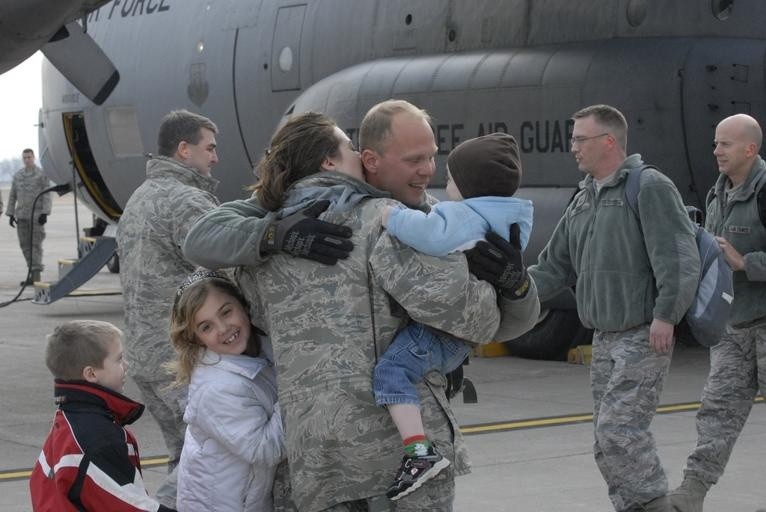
<box><xmin>182</xmin><ymin>98</ymin><xmax>542</xmax><ymax>511</ymax></box>
<box><xmin>114</xmin><ymin>108</ymin><xmax>266</xmax><ymax>511</ymax></box>
<box><xmin>28</xmin><ymin>319</ymin><xmax>175</xmax><ymax>511</ymax></box>
<box><xmin>667</xmin><ymin>113</ymin><xmax>765</xmax><ymax>511</ymax></box>
<box><xmin>249</xmin><ymin>110</ymin><xmax>504</xmax><ymax>510</ymax></box>
<box><xmin>525</xmin><ymin>103</ymin><xmax>703</xmax><ymax>511</ymax></box>
<box><xmin>370</xmin><ymin>133</ymin><xmax>534</xmax><ymax>501</ymax></box>
<box><xmin>6</xmin><ymin>147</ymin><xmax>52</xmax><ymax>289</ymax></box>
<box><xmin>176</xmin><ymin>267</ymin><xmax>289</xmax><ymax>510</ymax></box>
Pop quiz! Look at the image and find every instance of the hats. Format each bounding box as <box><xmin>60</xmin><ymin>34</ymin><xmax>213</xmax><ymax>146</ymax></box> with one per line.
<box><xmin>448</xmin><ymin>133</ymin><xmax>522</xmax><ymax>199</ymax></box>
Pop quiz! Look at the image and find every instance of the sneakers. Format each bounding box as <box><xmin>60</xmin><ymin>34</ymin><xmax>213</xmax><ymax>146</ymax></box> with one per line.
<box><xmin>386</xmin><ymin>441</ymin><xmax>451</xmax><ymax>500</ymax></box>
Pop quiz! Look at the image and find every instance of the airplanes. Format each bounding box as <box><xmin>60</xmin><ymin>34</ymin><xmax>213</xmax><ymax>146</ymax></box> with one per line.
<box><xmin>0</xmin><ymin>0</ymin><xmax>765</xmax><ymax>360</ymax></box>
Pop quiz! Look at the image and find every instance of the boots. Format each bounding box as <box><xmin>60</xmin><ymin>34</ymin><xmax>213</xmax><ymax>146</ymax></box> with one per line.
<box><xmin>666</xmin><ymin>476</ymin><xmax>707</xmax><ymax>512</ymax></box>
<box><xmin>21</xmin><ymin>270</ymin><xmax>40</xmax><ymax>286</ymax></box>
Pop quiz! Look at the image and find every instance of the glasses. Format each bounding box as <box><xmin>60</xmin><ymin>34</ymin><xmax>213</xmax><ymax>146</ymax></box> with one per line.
<box><xmin>570</xmin><ymin>133</ymin><xmax>608</xmax><ymax>145</ymax></box>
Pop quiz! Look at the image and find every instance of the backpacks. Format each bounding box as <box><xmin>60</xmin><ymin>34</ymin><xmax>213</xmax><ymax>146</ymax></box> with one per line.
<box><xmin>625</xmin><ymin>165</ymin><xmax>735</xmax><ymax>347</ymax></box>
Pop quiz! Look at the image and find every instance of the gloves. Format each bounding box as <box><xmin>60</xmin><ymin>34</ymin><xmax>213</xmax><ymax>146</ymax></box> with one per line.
<box><xmin>463</xmin><ymin>223</ymin><xmax>530</xmax><ymax>299</ymax></box>
<box><xmin>38</xmin><ymin>214</ymin><xmax>46</xmax><ymax>225</ymax></box>
<box><xmin>9</xmin><ymin>215</ymin><xmax>17</xmax><ymax>227</ymax></box>
<box><xmin>260</xmin><ymin>200</ymin><xmax>353</xmax><ymax>265</ymax></box>
